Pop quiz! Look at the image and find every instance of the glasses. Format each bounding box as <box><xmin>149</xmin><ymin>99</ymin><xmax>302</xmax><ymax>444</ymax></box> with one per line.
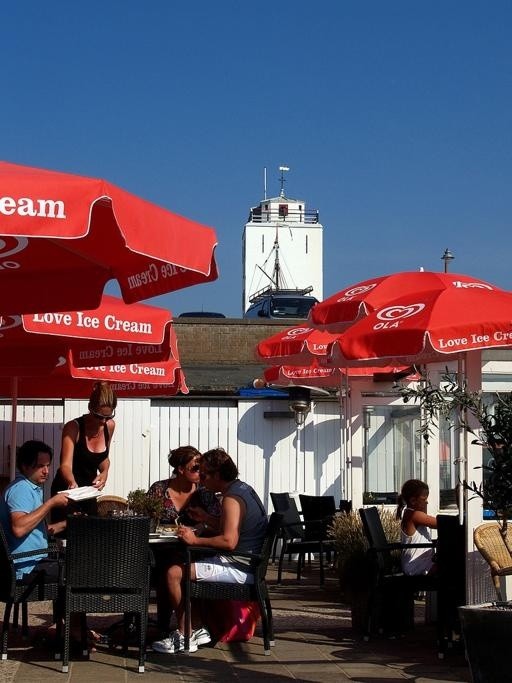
<box><xmin>184</xmin><ymin>464</ymin><xmax>199</xmax><ymax>472</ymax></box>
<box><xmin>90</xmin><ymin>408</ymin><xmax>116</xmax><ymax>421</ymax></box>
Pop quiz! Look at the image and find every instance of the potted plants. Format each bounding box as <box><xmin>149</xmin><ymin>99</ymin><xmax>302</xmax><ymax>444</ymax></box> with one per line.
<box><xmin>126</xmin><ymin>490</ymin><xmax>171</xmax><ymax>533</ymax></box>
<box><xmin>392</xmin><ymin>366</ymin><xmax>512</xmax><ymax>683</ymax></box>
<box><xmin>326</xmin><ymin>509</ymin><xmax>415</xmax><ymax>639</ymax></box>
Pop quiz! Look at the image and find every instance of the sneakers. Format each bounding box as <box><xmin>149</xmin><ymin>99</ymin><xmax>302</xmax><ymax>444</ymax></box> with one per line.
<box><xmin>152</xmin><ymin>629</ymin><xmax>198</xmax><ymax>653</ymax></box>
<box><xmin>191</xmin><ymin>628</ymin><xmax>211</xmax><ymax>645</ymax></box>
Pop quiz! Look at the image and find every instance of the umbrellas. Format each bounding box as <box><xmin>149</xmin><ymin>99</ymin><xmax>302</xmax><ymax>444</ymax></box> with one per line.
<box><xmin>325</xmin><ymin>285</ymin><xmax>512</xmax><ymax>552</ymax></box>
<box><xmin>1</xmin><ymin>160</ymin><xmax>219</xmax><ymax>316</ymax></box>
<box><xmin>0</xmin><ymin>291</ymin><xmax>181</xmax><ymax>363</ymax></box>
<box><xmin>0</xmin><ymin>361</ymin><xmax>189</xmax><ymax>485</ymax></box>
<box><xmin>255</xmin><ymin>321</ymin><xmax>465</xmax><ymax>499</ymax></box>
<box><xmin>262</xmin><ymin>359</ymin><xmax>431</xmax><ymax>515</ymax></box>
<box><xmin>306</xmin><ymin>268</ymin><xmax>508</xmax><ymax>483</ymax></box>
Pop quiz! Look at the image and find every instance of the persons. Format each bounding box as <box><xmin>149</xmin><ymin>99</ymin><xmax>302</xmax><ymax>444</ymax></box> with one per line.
<box><xmin>143</xmin><ymin>446</ymin><xmax>202</xmax><ymax>639</ymax></box>
<box><xmin>0</xmin><ymin>439</ymin><xmax>101</xmax><ymax>653</ymax></box>
<box><xmin>151</xmin><ymin>447</ymin><xmax>268</xmax><ymax>654</ymax></box>
<box><xmin>50</xmin><ymin>380</ymin><xmax>118</xmax><ymax>537</ymax></box>
<box><xmin>395</xmin><ymin>479</ymin><xmax>436</xmax><ymax>592</ymax></box>
<box><xmin>188</xmin><ymin>487</ymin><xmax>263</xmax><ymax>645</ymax></box>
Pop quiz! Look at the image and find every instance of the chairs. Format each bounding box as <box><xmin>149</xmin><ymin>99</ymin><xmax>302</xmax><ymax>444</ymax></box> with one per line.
<box><xmin>440</xmin><ymin>489</ymin><xmax>459</xmax><ymax>509</ymax></box>
<box><xmin>0</xmin><ymin>496</ymin><xmax>88</xmax><ymax>661</ymax></box>
<box><xmin>472</xmin><ymin>522</ymin><xmax>512</xmax><ymax>601</ymax></box>
<box><xmin>272</xmin><ymin>492</ymin><xmax>399</xmax><ymax>592</ymax></box>
<box><xmin>183</xmin><ymin>513</ymin><xmax>285</xmax><ymax>656</ymax></box>
<box><xmin>435</xmin><ymin>515</ymin><xmax>465</xmax><ymax>659</ymax></box>
<box><xmin>55</xmin><ymin>514</ymin><xmax>151</xmax><ymax>673</ymax></box>
<box><xmin>96</xmin><ymin>495</ymin><xmax>129</xmax><ymax>518</ymax></box>
<box><xmin>360</xmin><ymin>507</ymin><xmax>436</xmax><ymax>640</ymax></box>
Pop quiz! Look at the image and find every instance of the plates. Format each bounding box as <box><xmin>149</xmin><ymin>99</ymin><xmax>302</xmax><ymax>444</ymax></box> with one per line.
<box><xmin>57</xmin><ymin>486</ymin><xmax>105</xmax><ymax>502</ymax></box>
<box><xmin>149</xmin><ymin>529</ymin><xmax>197</xmax><ymax>539</ymax></box>
